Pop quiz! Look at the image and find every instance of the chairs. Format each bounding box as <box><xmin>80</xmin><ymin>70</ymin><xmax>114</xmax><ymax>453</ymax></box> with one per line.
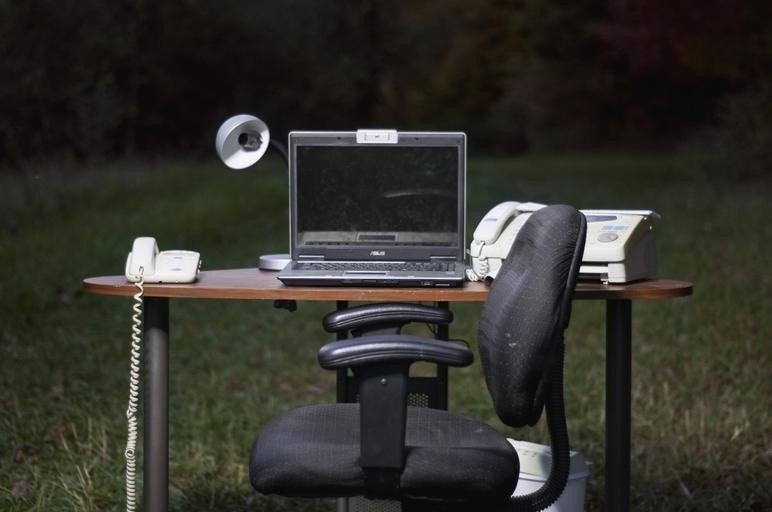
<box><xmin>249</xmin><ymin>202</ymin><xmax>587</xmax><ymax>512</ymax></box>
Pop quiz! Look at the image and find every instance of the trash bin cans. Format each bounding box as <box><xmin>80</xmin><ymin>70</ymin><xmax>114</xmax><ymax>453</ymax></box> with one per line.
<box><xmin>504</xmin><ymin>436</ymin><xmax>595</xmax><ymax>512</ymax></box>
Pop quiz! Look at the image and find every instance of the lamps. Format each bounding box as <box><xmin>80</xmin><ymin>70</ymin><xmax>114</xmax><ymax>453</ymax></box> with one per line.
<box><xmin>215</xmin><ymin>111</ymin><xmax>293</xmax><ymax>273</ymax></box>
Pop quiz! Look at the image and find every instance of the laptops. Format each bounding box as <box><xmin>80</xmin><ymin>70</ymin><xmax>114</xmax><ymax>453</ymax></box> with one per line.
<box><xmin>276</xmin><ymin>129</ymin><xmax>468</xmax><ymax>287</ymax></box>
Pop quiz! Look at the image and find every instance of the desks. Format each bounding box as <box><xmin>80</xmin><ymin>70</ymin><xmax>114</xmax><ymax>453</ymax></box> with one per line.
<box><xmin>81</xmin><ymin>259</ymin><xmax>695</xmax><ymax>512</ymax></box>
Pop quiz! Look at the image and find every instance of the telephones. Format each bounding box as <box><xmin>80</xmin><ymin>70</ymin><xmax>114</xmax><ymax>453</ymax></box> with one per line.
<box><xmin>124</xmin><ymin>237</ymin><xmax>201</xmax><ymax>284</ymax></box>
